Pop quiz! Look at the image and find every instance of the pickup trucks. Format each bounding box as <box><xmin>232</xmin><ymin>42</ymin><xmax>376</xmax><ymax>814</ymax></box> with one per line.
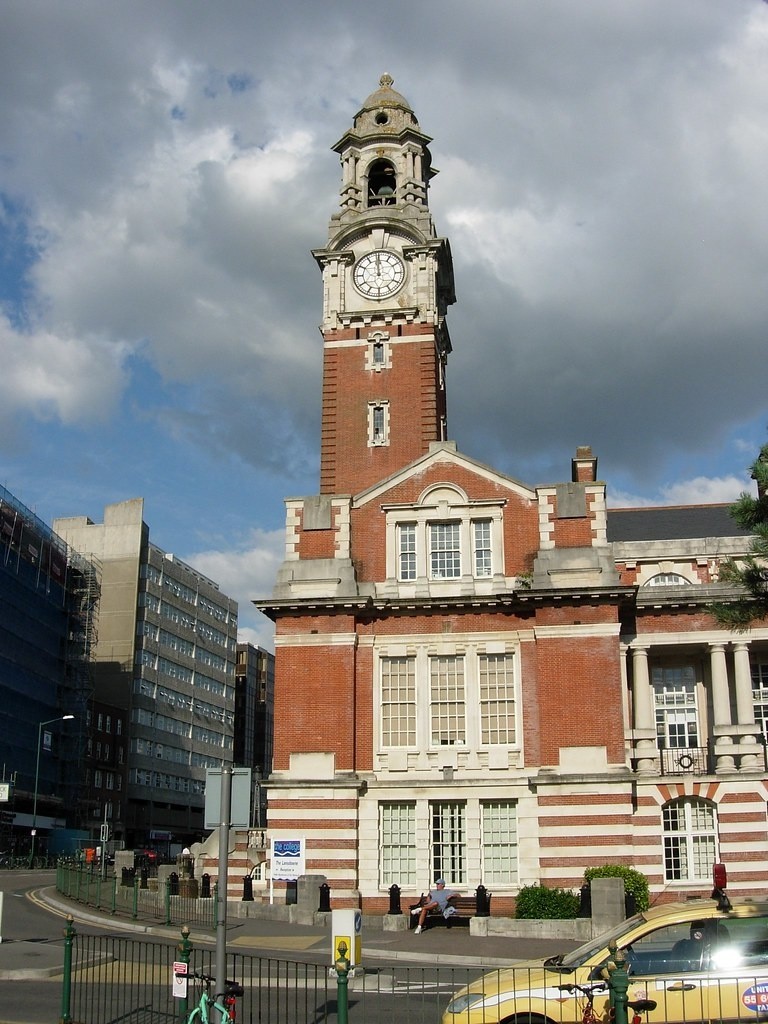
<box><xmin>109</xmin><ymin>848</ymin><xmax>149</xmax><ymax>866</ymax></box>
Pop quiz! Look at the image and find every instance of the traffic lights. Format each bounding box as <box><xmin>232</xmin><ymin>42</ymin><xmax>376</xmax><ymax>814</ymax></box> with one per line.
<box><xmin>108</xmin><ymin>825</ymin><xmax>113</xmax><ymax>840</ymax></box>
<box><xmin>100</xmin><ymin>825</ymin><xmax>108</xmax><ymax>841</ymax></box>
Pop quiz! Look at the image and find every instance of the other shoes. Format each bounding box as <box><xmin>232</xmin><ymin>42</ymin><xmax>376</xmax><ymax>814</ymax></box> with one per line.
<box><xmin>414</xmin><ymin>927</ymin><xmax>421</xmax><ymax>934</ymax></box>
<box><xmin>411</xmin><ymin>909</ymin><xmax>420</xmax><ymax>914</ymax></box>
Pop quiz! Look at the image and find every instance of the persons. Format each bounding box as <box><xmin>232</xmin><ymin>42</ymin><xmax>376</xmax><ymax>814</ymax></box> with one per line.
<box><xmin>411</xmin><ymin>879</ymin><xmax>461</xmax><ymax>935</ymax></box>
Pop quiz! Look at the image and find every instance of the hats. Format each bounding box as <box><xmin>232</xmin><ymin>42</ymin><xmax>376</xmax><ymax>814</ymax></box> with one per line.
<box><xmin>436</xmin><ymin>878</ymin><xmax>445</xmax><ymax>887</ymax></box>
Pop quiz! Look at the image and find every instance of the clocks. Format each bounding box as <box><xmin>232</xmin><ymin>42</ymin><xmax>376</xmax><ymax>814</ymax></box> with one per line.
<box><xmin>350</xmin><ymin>249</ymin><xmax>408</xmax><ymax>301</ymax></box>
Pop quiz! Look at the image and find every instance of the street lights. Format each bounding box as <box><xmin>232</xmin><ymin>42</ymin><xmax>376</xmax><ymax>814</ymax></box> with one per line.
<box><xmin>27</xmin><ymin>715</ymin><xmax>74</xmax><ymax>870</ymax></box>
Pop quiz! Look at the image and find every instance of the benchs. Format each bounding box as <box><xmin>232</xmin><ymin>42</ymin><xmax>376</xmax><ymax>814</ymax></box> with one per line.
<box><xmin>408</xmin><ymin>892</ymin><xmax>492</xmax><ymax>930</ymax></box>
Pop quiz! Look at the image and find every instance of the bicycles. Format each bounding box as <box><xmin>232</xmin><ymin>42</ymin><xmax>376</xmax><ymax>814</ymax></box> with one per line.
<box><xmin>175</xmin><ymin>968</ymin><xmax>244</xmax><ymax>1024</ymax></box>
<box><xmin>0</xmin><ymin>850</ymin><xmax>85</xmax><ymax>868</ymax></box>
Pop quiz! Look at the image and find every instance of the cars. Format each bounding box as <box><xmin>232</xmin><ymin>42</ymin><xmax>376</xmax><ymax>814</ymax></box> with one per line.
<box><xmin>442</xmin><ymin>864</ymin><xmax>768</xmax><ymax>1024</ymax></box>
<box><xmin>145</xmin><ymin>850</ymin><xmax>161</xmax><ymax>862</ymax></box>
